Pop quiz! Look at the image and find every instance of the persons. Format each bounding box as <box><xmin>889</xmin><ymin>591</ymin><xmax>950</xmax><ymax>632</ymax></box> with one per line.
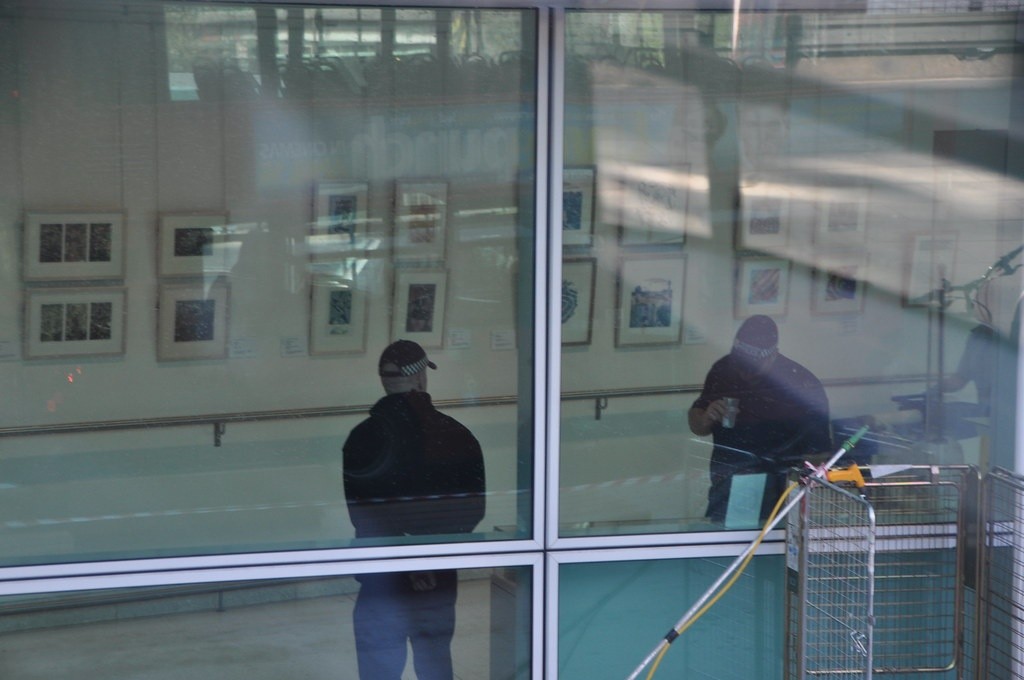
<box><xmin>944</xmin><ymin>282</ymin><xmax>1002</xmax><ymax>406</ymax></box>
<box><xmin>689</xmin><ymin>311</ymin><xmax>832</xmax><ymax>520</ymax></box>
<box><xmin>339</xmin><ymin>338</ymin><xmax>487</xmax><ymax>680</ymax></box>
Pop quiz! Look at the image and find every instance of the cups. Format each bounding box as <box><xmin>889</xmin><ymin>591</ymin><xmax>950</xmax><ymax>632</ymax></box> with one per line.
<box><xmin>721</xmin><ymin>397</ymin><xmax>739</xmax><ymax>429</ymax></box>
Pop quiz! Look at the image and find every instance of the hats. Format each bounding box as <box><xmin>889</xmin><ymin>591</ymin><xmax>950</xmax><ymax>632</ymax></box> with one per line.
<box><xmin>728</xmin><ymin>315</ymin><xmax>778</xmax><ymax>371</ymax></box>
<box><xmin>376</xmin><ymin>340</ymin><xmax>437</xmax><ymax>377</ymax></box>
